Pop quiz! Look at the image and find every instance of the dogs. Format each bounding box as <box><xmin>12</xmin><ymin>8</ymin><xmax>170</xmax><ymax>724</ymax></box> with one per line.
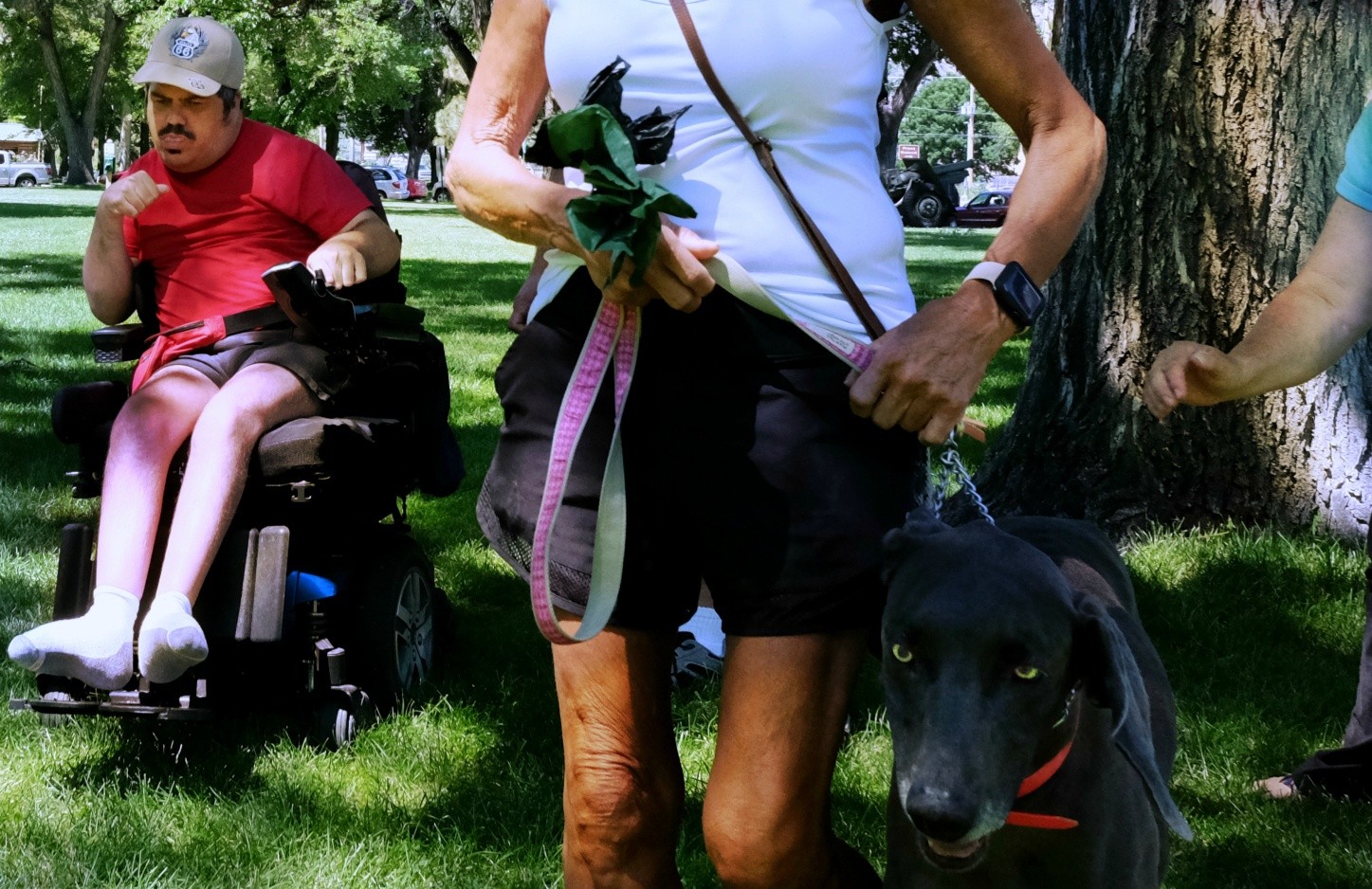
<box><xmin>874</xmin><ymin>515</ymin><xmax>1182</xmax><ymax>889</ymax></box>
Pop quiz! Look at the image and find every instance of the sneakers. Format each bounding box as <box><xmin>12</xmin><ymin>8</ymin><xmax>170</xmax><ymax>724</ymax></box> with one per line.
<box><xmin>669</xmin><ymin>632</ymin><xmax>726</xmax><ymax>703</ymax></box>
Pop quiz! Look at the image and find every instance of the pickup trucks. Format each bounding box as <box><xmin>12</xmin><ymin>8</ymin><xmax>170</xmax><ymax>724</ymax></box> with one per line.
<box><xmin>0</xmin><ymin>150</ymin><xmax>54</xmax><ymax>188</ymax></box>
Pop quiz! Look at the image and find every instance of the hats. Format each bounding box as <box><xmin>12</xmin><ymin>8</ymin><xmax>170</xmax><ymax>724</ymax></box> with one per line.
<box><xmin>132</xmin><ymin>15</ymin><xmax>244</xmax><ymax>96</ymax></box>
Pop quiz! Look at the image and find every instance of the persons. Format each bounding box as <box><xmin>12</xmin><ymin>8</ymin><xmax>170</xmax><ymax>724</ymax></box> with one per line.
<box><xmin>507</xmin><ymin>167</ymin><xmax>587</xmax><ymax>333</ymax></box>
<box><xmin>1140</xmin><ymin>99</ymin><xmax>1372</xmax><ymax>420</ymax></box>
<box><xmin>442</xmin><ymin>0</ymin><xmax>1109</xmax><ymax>889</ymax></box>
<box><xmin>7</xmin><ymin>18</ymin><xmax>400</xmax><ymax>690</ymax></box>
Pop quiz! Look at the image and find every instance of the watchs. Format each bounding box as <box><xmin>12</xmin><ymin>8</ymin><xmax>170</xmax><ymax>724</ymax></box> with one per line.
<box><xmin>962</xmin><ymin>261</ymin><xmax>1046</xmax><ymax>337</ymax></box>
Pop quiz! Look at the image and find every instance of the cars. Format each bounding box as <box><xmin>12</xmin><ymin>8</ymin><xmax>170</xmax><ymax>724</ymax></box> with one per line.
<box><xmin>361</xmin><ymin>163</ymin><xmax>431</xmax><ymax>201</ymax></box>
<box><xmin>431</xmin><ymin>176</ymin><xmax>453</xmax><ymax>203</ymax></box>
<box><xmin>950</xmin><ymin>191</ymin><xmax>1013</xmax><ymax>228</ymax></box>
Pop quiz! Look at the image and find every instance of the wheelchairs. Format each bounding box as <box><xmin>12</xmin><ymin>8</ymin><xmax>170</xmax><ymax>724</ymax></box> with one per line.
<box><xmin>9</xmin><ymin>159</ymin><xmax>440</xmax><ymax>757</ymax></box>
